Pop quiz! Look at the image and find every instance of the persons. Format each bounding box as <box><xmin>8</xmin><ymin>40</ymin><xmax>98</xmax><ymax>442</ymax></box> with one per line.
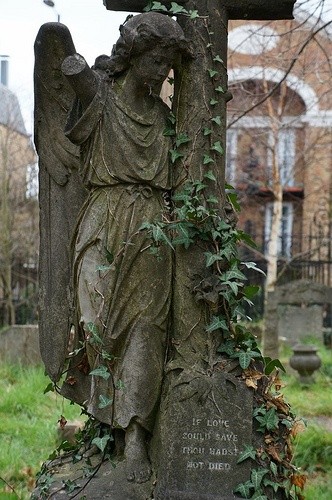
<box><xmin>61</xmin><ymin>11</ymin><xmax>188</xmax><ymax>483</ymax></box>
